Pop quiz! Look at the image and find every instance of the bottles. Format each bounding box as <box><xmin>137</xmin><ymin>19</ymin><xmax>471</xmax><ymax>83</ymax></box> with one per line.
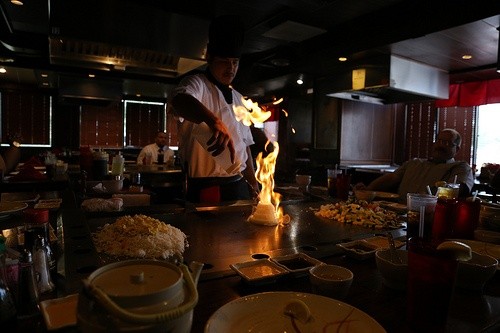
<box><xmin>337</xmin><ymin>173</ymin><xmax>355</xmax><ymax>198</ymax></box>
<box><xmin>13</xmin><ymin>234</ymin><xmax>56</xmax><ymax>319</ymax></box>
<box><xmin>79</xmin><ymin>145</ymin><xmax>125</xmax><ymax>180</ymax></box>
<box><xmin>45</xmin><ymin>152</ymin><xmax>68</xmax><ymax>178</ymax></box>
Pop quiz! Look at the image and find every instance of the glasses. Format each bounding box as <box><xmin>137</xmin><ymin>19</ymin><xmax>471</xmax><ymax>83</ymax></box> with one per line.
<box><xmin>435</xmin><ymin>136</ymin><xmax>458</xmax><ymax>148</ymax></box>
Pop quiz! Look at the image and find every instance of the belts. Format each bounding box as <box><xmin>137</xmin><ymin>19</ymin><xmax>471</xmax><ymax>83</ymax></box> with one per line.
<box><xmin>187</xmin><ymin>177</ymin><xmax>241</xmax><ymax>183</ymax></box>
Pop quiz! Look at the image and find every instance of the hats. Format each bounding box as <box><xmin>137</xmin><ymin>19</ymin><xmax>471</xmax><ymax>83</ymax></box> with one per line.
<box><xmin>207</xmin><ymin>36</ymin><xmax>248</xmax><ymax>58</ymax></box>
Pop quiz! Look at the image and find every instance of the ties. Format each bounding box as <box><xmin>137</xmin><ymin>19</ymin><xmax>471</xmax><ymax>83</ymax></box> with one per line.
<box><xmin>158</xmin><ymin>148</ymin><xmax>164</xmax><ymax>171</ymax></box>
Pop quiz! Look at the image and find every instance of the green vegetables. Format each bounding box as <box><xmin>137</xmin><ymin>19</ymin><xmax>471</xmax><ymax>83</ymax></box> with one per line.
<box><xmin>329</xmin><ymin>199</ymin><xmax>398</xmax><ymax>229</ymax></box>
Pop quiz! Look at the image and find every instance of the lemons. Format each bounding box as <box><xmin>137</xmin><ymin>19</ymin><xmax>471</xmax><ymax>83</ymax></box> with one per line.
<box><xmin>436</xmin><ymin>241</ymin><xmax>472</xmax><ymax>261</ymax></box>
<box><xmin>283</xmin><ymin>299</ymin><xmax>311</xmax><ymax>323</ymax></box>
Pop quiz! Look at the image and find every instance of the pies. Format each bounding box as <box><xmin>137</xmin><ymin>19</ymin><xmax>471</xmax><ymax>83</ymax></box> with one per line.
<box><xmin>0</xmin><ymin>201</ymin><xmax>28</xmax><ymax>213</ymax></box>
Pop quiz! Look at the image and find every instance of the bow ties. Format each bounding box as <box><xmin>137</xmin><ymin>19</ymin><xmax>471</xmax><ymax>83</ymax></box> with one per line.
<box><xmin>427</xmin><ymin>158</ymin><xmax>444</xmax><ymax>165</ymax></box>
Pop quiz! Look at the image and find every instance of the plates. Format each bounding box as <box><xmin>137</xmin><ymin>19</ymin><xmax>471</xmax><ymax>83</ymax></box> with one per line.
<box><xmin>0</xmin><ymin>192</ymin><xmax>40</xmax><ymax>214</ymax></box>
<box><xmin>34</xmin><ymin>199</ymin><xmax>62</xmax><ymax>210</ymax></box>
<box><xmin>337</xmin><ymin>237</ymin><xmax>406</xmax><ymax>255</ymax></box>
<box><xmin>204</xmin><ymin>291</ymin><xmax>387</xmax><ymax>333</ymax></box>
<box><xmin>40</xmin><ymin>292</ymin><xmax>79</xmax><ymax>332</ymax></box>
<box><xmin>228</xmin><ymin>253</ymin><xmax>328</xmax><ymax>288</ymax></box>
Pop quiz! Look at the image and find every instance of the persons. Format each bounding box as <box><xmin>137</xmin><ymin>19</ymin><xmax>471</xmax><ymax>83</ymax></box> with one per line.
<box><xmin>353</xmin><ymin>129</ymin><xmax>474</xmax><ymax>198</ymax></box>
<box><xmin>166</xmin><ymin>39</ymin><xmax>260</xmax><ymax>206</ymax></box>
<box><xmin>137</xmin><ymin>132</ymin><xmax>174</xmax><ymax>164</ymax></box>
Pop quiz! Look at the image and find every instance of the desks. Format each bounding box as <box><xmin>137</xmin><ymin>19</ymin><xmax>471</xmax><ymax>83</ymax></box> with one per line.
<box><xmin>111</xmin><ymin>162</ymin><xmax>182</xmax><ymax>185</ymax></box>
<box><xmin>3</xmin><ymin>164</ymin><xmax>69</xmax><ymax>191</ymax></box>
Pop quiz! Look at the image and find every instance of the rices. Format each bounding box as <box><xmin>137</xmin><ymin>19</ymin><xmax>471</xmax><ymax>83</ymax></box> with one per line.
<box><xmin>90</xmin><ymin>213</ymin><xmax>188</xmax><ymax>260</ymax></box>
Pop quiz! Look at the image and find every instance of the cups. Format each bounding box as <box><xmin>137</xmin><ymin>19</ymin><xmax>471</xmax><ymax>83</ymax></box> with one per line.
<box><xmin>407</xmin><ymin>192</ymin><xmax>437</xmax><ymax>247</ymax></box>
<box><xmin>327</xmin><ymin>169</ymin><xmax>343</xmax><ymax>193</ymax></box>
<box><xmin>432</xmin><ymin>202</ymin><xmax>449</xmax><ymax>242</ymax></box>
<box><xmin>167</xmin><ymin>156</ymin><xmax>176</xmax><ymax>169</ymax></box>
<box><xmin>453</xmin><ymin>199</ymin><xmax>480</xmax><ymax>238</ymax></box>
<box><xmin>439</xmin><ymin>185</ymin><xmax>460</xmax><ymax>199</ymax></box>
<box><xmin>133</xmin><ymin>173</ymin><xmax>140</xmax><ymax>186</ymax></box>
<box><xmin>355</xmin><ymin>189</ymin><xmax>376</xmax><ymax>202</ymax></box>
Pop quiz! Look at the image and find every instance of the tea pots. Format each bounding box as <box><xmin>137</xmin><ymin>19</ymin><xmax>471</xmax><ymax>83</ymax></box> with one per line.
<box><xmin>76</xmin><ymin>258</ymin><xmax>204</xmax><ymax>333</ymax></box>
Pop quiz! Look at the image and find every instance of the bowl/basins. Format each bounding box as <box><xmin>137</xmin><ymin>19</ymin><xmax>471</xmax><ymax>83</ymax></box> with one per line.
<box><xmin>473</xmin><ymin>229</ymin><xmax>500</xmax><ymax>243</ymax></box>
<box><xmin>309</xmin><ymin>264</ymin><xmax>354</xmax><ymax>299</ymax></box>
<box><xmin>447</xmin><ymin>294</ymin><xmax>494</xmax><ymax>329</ymax></box>
<box><xmin>446</xmin><ymin>247</ymin><xmax>499</xmax><ymax>290</ymax></box>
<box><xmin>375</xmin><ymin>192</ymin><xmax>401</xmax><ymax>203</ymax></box>
<box><xmin>376</xmin><ymin>249</ymin><xmax>408</xmax><ymax>278</ymax></box>
<box><xmin>378</xmin><ymin>201</ymin><xmax>407</xmax><ymax>214</ymax></box>
<box><xmin>296</xmin><ymin>175</ymin><xmax>312</xmax><ymax>190</ymax></box>
